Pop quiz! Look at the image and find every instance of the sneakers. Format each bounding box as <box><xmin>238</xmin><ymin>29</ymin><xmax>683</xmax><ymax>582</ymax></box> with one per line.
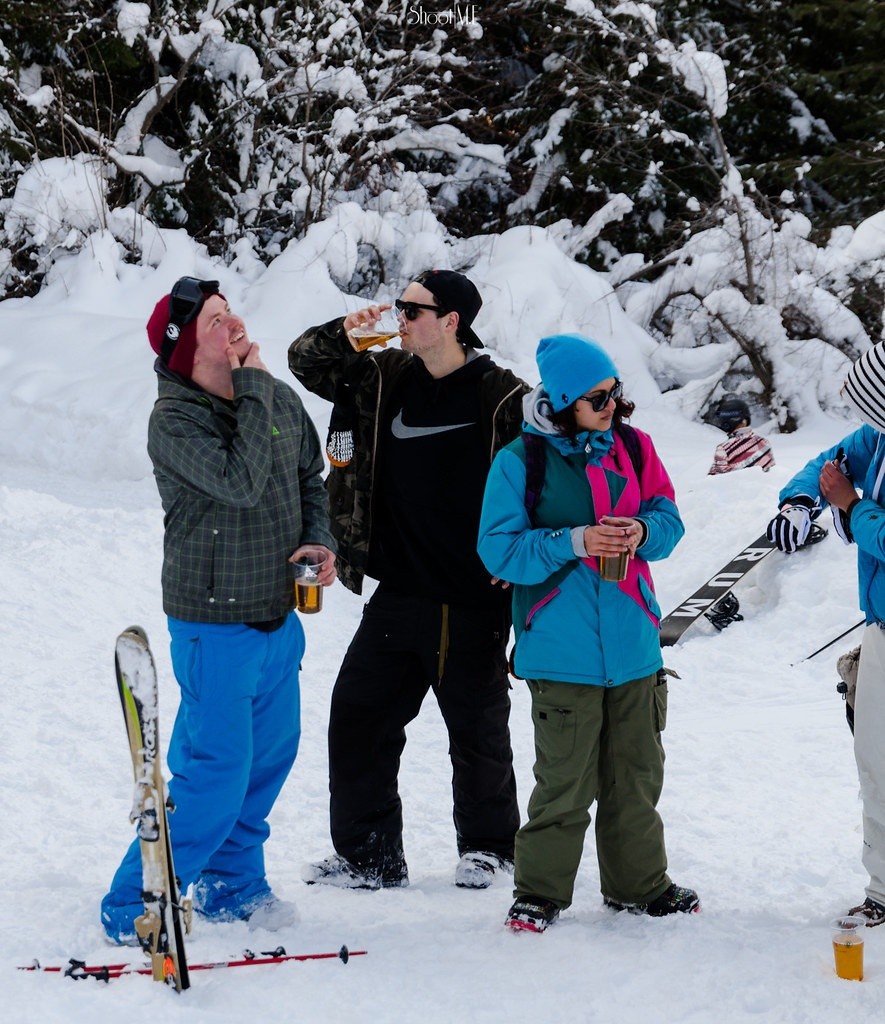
<box><xmin>603</xmin><ymin>881</ymin><xmax>698</xmax><ymax>918</ymax></box>
<box><xmin>503</xmin><ymin>896</ymin><xmax>561</xmax><ymax>933</ymax></box>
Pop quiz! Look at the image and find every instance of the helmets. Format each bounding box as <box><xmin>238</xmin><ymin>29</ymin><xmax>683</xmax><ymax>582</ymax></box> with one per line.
<box><xmin>711</xmin><ymin>399</ymin><xmax>751</xmax><ymax>435</ymax></box>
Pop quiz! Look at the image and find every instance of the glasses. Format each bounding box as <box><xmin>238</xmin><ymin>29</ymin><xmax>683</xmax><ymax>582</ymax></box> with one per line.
<box><xmin>169</xmin><ymin>275</ymin><xmax>219</xmax><ymax>324</ymax></box>
<box><xmin>395</xmin><ymin>298</ymin><xmax>444</xmax><ymax>321</ymax></box>
<box><xmin>578</xmin><ymin>377</ymin><xmax>622</xmax><ymax>412</ymax></box>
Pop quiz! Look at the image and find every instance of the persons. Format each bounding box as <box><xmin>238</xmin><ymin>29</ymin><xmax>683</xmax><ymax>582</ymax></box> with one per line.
<box><xmin>767</xmin><ymin>339</ymin><xmax>885</xmax><ymax>925</ymax></box>
<box><xmin>477</xmin><ymin>334</ymin><xmax>701</xmax><ymax>935</ymax></box>
<box><xmin>289</xmin><ymin>270</ymin><xmax>533</xmax><ymax>890</ymax></box>
<box><xmin>99</xmin><ymin>276</ymin><xmax>338</xmax><ymax>949</ymax></box>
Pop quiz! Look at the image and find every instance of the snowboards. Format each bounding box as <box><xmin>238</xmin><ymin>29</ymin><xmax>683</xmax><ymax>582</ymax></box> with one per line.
<box><xmin>659</xmin><ymin>502</ymin><xmax>827</xmax><ymax>650</ymax></box>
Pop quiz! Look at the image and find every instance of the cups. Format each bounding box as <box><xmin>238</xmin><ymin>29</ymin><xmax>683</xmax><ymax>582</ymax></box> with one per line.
<box><xmin>829</xmin><ymin>915</ymin><xmax>866</xmax><ymax>981</ymax></box>
<box><xmin>599</xmin><ymin>517</ymin><xmax>634</xmax><ymax>581</ymax></box>
<box><xmin>293</xmin><ymin>550</ymin><xmax>328</xmax><ymax>613</ymax></box>
<box><xmin>347</xmin><ymin>305</ymin><xmax>408</xmax><ymax>353</ymax></box>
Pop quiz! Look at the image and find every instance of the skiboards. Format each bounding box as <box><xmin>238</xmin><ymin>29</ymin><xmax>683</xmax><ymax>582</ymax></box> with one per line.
<box><xmin>111</xmin><ymin>622</ymin><xmax>194</xmax><ymax>993</ymax></box>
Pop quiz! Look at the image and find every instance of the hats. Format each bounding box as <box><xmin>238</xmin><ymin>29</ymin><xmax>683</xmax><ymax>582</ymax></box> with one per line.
<box><xmin>536</xmin><ymin>334</ymin><xmax>619</xmax><ymax>413</ymax></box>
<box><xmin>147</xmin><ymin>292</ymin><xmax>226</xmax><ymax>381</ymax></box>
<box><xmin>413</xmin><ymin>269</ymin><xmax>484</xmax><ymax>350</ymax></box>
<box><xmin>840</xmin><ymin>340</ymin><xmax>885</xmax><ymax>434</ymax></box>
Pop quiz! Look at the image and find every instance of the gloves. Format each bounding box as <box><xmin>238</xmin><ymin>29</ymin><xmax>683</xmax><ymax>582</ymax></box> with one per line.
<box><xmin>765</xmin><ymin>494</ymin><xmax>822</xmax><ymax>554</ymax></box>
<box><xmin>829</xmin><ymin>446</ymin><xmax>855</xmax><ymax>546</ymax></box>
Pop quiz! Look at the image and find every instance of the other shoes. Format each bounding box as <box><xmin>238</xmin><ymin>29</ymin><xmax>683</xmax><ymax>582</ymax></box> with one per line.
<box><xmin>839</xmin><ymin>897</ymin><xmax>885</xmax><ymax>929</ymax></box>
<box><xmin>105</xmin><ymin>929</ymin><xmax>145</xmax><ymax>951</ymax></box>
<box><xmin>238</xmin><ymin>897</ymin><xmax>297</xmax><ymax>931</ymax></box>
<box><xmin>300</xmin><ymin>851</ymin><xmax>409</xmax><ymax>889</ymax></box>
<box><xmin>457</xmin><ymin>850</ymin><xmax>500</xmax><ymax>889</ymax></box>
